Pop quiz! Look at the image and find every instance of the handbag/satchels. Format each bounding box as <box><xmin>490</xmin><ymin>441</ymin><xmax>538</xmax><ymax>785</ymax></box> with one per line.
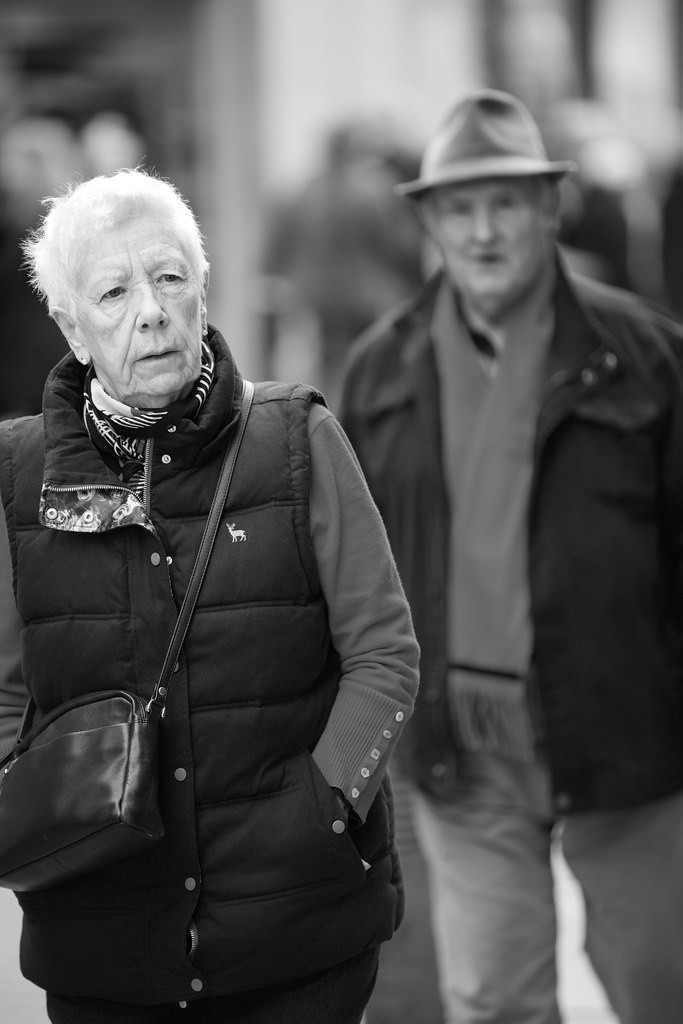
<box><xmin>1</xmin><ymin>688</ymin><xmax>152</xmax><ymax>898</ymax></box>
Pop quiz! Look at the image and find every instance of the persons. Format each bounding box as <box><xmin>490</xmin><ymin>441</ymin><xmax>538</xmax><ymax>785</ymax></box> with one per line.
<box><xmin>337</xmin><ymin>87</ymin><xmax>683</xmax><ymax>1024</ymax></box>
<box><xmin>0</xmin><ymin>164</ymin><xmax>419</xmax><ymax>1024</ymax></box>
<box><xmin>0</xmin><ymin>111</ymin><xmax>149</xmax><ymax>418</ymax></box>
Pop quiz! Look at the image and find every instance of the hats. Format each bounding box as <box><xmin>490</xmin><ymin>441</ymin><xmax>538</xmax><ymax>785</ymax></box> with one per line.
<box><xmin>396</xmin><ymin>89</ymin><xmax>572</xmax><ymax>196</ymax></box>
